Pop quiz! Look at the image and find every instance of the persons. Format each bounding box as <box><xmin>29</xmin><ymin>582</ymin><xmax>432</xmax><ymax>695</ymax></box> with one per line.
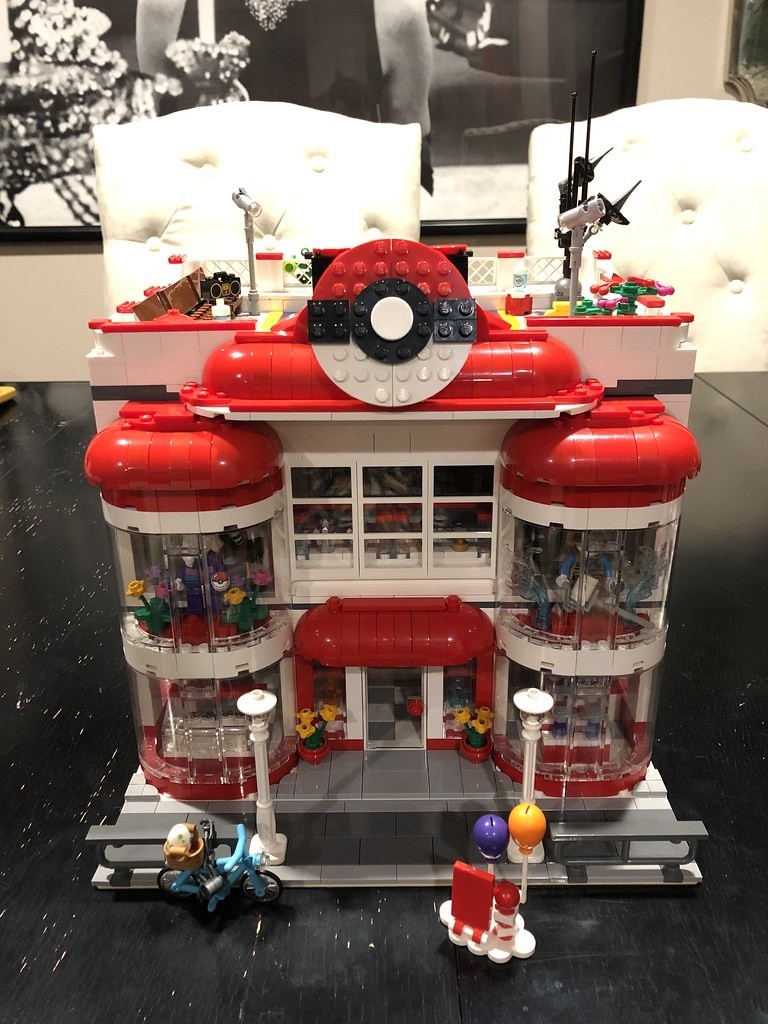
<box><xmin>135</xmin><ymin>0</ymin><xmax>434</xmax><ymax>200</ymax></box>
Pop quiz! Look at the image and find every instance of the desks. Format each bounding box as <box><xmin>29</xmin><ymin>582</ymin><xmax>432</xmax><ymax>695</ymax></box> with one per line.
<box><xmin>0</xmin><ymin>372</ymin><xmax>768</xmax><ymax>1024</ymax></box>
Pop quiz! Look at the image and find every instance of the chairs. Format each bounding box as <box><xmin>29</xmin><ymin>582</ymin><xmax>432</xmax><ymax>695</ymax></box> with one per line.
<box><xmin>461</xmin><ymin>118</ymin><xmax>565</xmax><ymax>165</ymax></box>
<box><xmin>92</xmin><ymin>101</ymin><xmax>421</xmax><ymax>313</ymax></box>
<box><xmin>525</xmin><ymin>98</ymin><xmax>765</xmax><ymax>373</ymax></box>
